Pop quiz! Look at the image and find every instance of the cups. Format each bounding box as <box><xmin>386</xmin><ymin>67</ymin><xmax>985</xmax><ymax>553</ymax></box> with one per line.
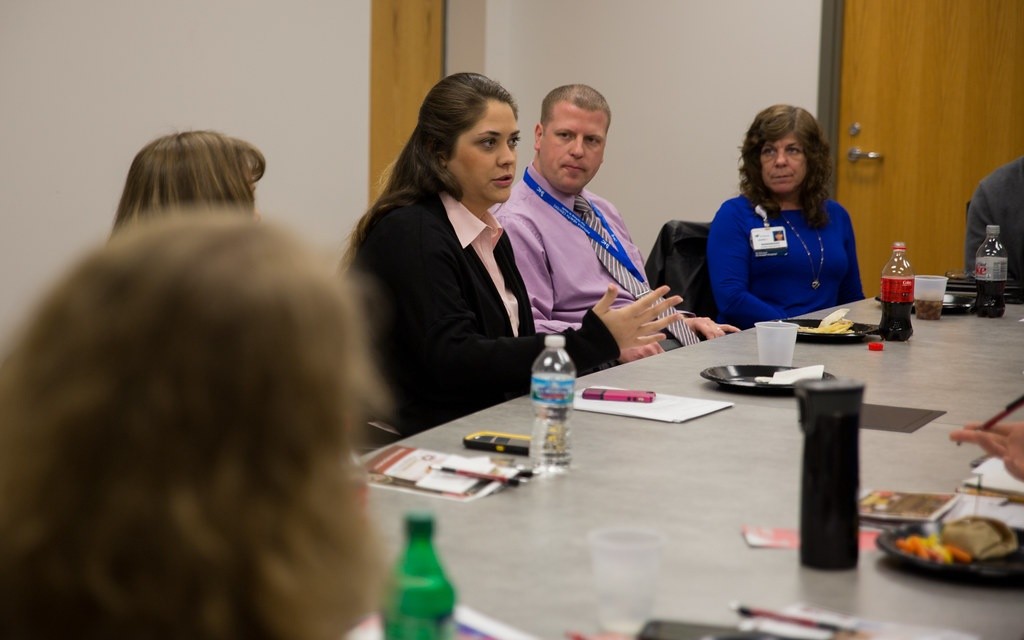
<box><xmin>795</xmin><ymin>378</ymin><xmax>866</xmax><ymax>571</ymax></box>
<box><xmin>754</xmin><ymin>321</ymin><xmax>799</xmax><ymax>367</ymax></box>
<box><xmin>914</xmin><ymin>275</ymin><xmax>948</xmax><ymax>319</ymax></box>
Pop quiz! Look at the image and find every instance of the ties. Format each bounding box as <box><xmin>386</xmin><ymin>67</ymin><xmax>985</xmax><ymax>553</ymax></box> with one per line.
<box><xmin>574</xmin><ymin>194</ymin><xmax>700</xmax><ymax>347</ymax></box>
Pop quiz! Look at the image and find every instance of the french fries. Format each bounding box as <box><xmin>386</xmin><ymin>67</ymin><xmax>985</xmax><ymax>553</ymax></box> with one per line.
<box><xmin>808</xmin><ymin>320</ymin><xmax>854</xmax><ymax>333</ymax></box>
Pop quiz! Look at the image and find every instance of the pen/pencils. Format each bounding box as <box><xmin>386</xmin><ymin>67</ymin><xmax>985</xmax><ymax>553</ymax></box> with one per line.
<box><xmin>516</xmin><ymin>471</ymin><xmax>539</xmax><ymax>477</ymax></box>
<box><xmin>738</xmin><ymin>607</ymin><xmax>855</xmax><ymax>634</ymax></box>
<box><xmin>956</xmin><ymin>395</ymin><xmax>1024</xmax><ymax>446</ymax></box>
<box><xmin>430</xmin><ymin>464</ymin><xmax>519</xmax><ymax>487</ymax></box>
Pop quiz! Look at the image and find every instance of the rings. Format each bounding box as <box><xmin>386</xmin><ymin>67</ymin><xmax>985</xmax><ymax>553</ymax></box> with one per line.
<box><xmin>717</xmin><ymin>327</ymin><xmax>721</xmax><ymax>331</ymax></box>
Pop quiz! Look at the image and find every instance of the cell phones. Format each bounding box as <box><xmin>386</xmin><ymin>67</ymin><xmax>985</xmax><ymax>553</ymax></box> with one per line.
<box><xmin>638</xmin><ymin>621</ymin><xmax>738</xmax><ymax>640</ymax></box>
<box><xmin>464</xmin><ymin>432</ymin><xmax>532</xmax><ymax>455</ymax></box>
<box><xmin>582</xmin><ymin>388</ymin><xmax>656</xmax><ymax>403</ymax></box>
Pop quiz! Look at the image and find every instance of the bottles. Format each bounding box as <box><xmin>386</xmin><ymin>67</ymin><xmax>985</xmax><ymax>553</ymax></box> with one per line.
<box><xmin>879</xmin><ymin>243</ymin><xmax>914</xmax><ymax>341</ymax></box>
<box><xmin>975</xmin><ymin>225</ymin><xmax>1008</xmax><ymax>318</ymax></box>
<box><xmin>383</xmin><ymin>511</ymin><xmax>454</xmax><ymax>640</ymax></box>
<box><xmin>531</xmin><ymin>335</ymin><xmax>578</xmax><ymax>473</ymax></box>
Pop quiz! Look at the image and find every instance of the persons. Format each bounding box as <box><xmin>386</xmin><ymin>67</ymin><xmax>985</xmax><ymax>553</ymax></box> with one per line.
<box><xmin>329</xmin><ymin>73</ymin><xmax>684</xmax><ymax>452</ymax></box>
<box><xmin>774</xmin><ymin>232</ymin><xmax>784</xmax><ymax>241</ymax></box>
<box><xmin>105</xmin><ymin>131</ymin><xmax>266</xmax><ymax>241</ymax></box>
<box><xmin>707</xmin><ymin>104</ymin><xmax>866</xmax><ymax>331</ymax></box>
<box><xmin>487</xmin><ymin>84</ymin><xmax>740</xmax><ymax>363</ymax></box>
<box><xmin>949</xmin><ymin>421</ymin><xmax>1024</xmax><ymax>481</ymax></box>
<box><xmin>0</xmin><ymin>213</ymin><xmax>390</xmax><ymax>639</ymax></box>
<box><xmin>964</xmin><ymin>156</ymin><xmax>1024</xmax><ymax>282</ymax></box>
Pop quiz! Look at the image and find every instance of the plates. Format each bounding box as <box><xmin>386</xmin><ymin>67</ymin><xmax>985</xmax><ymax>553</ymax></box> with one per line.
<box><xmin>780</xmin><ymin>319</ymin><xmax>879</xmax><ymax>342</ymax></box>
<box><xmin>873</xmin><ymin>293</ymin><xmax>977</xmax><ymax>314</ymax></box>
<box><xmin>876</xmin><ymin>521</ymin><xmax>1023</xmax><ymax>584</ymax></box>
<box><xmin>946</xmin><ymin>276</ymin><xmax>1023</xmax><ymax>302</ymax></box>
<box><xmin>699</xmin><ymin>364</ymin><xmax>836</xmax><ymax>396</ymax></box>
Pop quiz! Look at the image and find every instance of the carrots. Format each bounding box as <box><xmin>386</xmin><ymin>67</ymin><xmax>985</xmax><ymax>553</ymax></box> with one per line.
<box><xmin>896</xmin><ymin>537</ymin><xmax>973</xmax><ymax>563</ymax></box>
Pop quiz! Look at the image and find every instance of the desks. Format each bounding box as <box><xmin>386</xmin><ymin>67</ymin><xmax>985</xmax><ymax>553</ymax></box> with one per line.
<box><xmin>356</xmin><ymin>276</ymin><xmax>1024</xmax><ymax>640</ymax></box>
